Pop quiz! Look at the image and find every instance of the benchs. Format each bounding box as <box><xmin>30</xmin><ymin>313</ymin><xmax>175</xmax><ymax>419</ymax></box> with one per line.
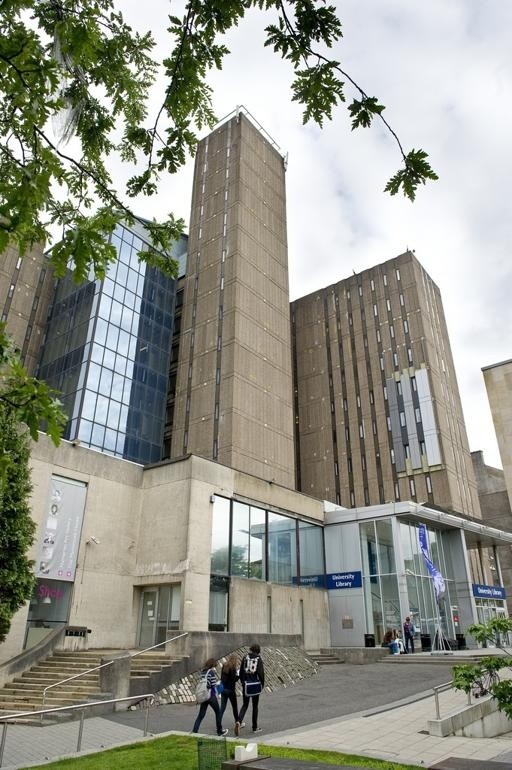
<box><xmin>222</xmin><ymin>754</ymin><xmax>511</xmax><ymax>770</ymax></box>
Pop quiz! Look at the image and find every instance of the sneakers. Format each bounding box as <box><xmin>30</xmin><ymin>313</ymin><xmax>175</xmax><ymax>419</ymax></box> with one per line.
<box><xmin>234</xmin><ymin>721</ymin><xmax>240</xmax><ymax>737</ymax></box>
<box><xmin>218</xmin><ymin>729</ymin><xmax>228</xmax><ymax>736</ymax></box>
<box><xmin>240</xmin><ymin>722</ymin><xmax>245</xmax><ymax>728</ymax></box>
<box><xmin>254</xmin><ymin>727</ymin><xmax>262</xmax><ymax>733</ymax></box>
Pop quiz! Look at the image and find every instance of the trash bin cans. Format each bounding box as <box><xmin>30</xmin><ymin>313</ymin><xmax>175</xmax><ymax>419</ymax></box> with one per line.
<box><xmin>456</xmin><ymin>634</ymin><xmax>466</xmax><ymax>650</ymax></box>
<box><xmin>364</xmin><ymin>633</ymin><xmax>375</xmax><ymax>647</ymax></box>
<box><xmin>420</xmin><ymin>634</ymin><xmax>432</xmax><ymax>652</ymax></box>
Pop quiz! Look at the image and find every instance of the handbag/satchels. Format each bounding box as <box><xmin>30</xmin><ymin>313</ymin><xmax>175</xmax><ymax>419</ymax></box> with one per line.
<box><xmin>215</xmin><ymin>681</ymin><xmax>224</xmax><ymax>694</ymax></box>
<box><xmin>245</xmin><ymin>681</ymin><xmax>262</xmax><ymax>697</ymax></box>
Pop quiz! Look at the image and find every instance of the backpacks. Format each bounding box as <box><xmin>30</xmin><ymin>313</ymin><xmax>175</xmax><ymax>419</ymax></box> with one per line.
<box><xmin>195</xmin><ymin>669</ymin><xmax>212</xmax><ymax>704</ymax></box>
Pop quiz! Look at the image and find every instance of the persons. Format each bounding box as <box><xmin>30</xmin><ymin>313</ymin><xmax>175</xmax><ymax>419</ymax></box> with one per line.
<box><xmin>381</xmin><ymin>617</ymin><xmax>415</xmax><ymax>656</ymax></box>
<box><xmin>192</xmin><ymin>644</ymin><xmax>264</xmax><ymax>736</ymax></box>
